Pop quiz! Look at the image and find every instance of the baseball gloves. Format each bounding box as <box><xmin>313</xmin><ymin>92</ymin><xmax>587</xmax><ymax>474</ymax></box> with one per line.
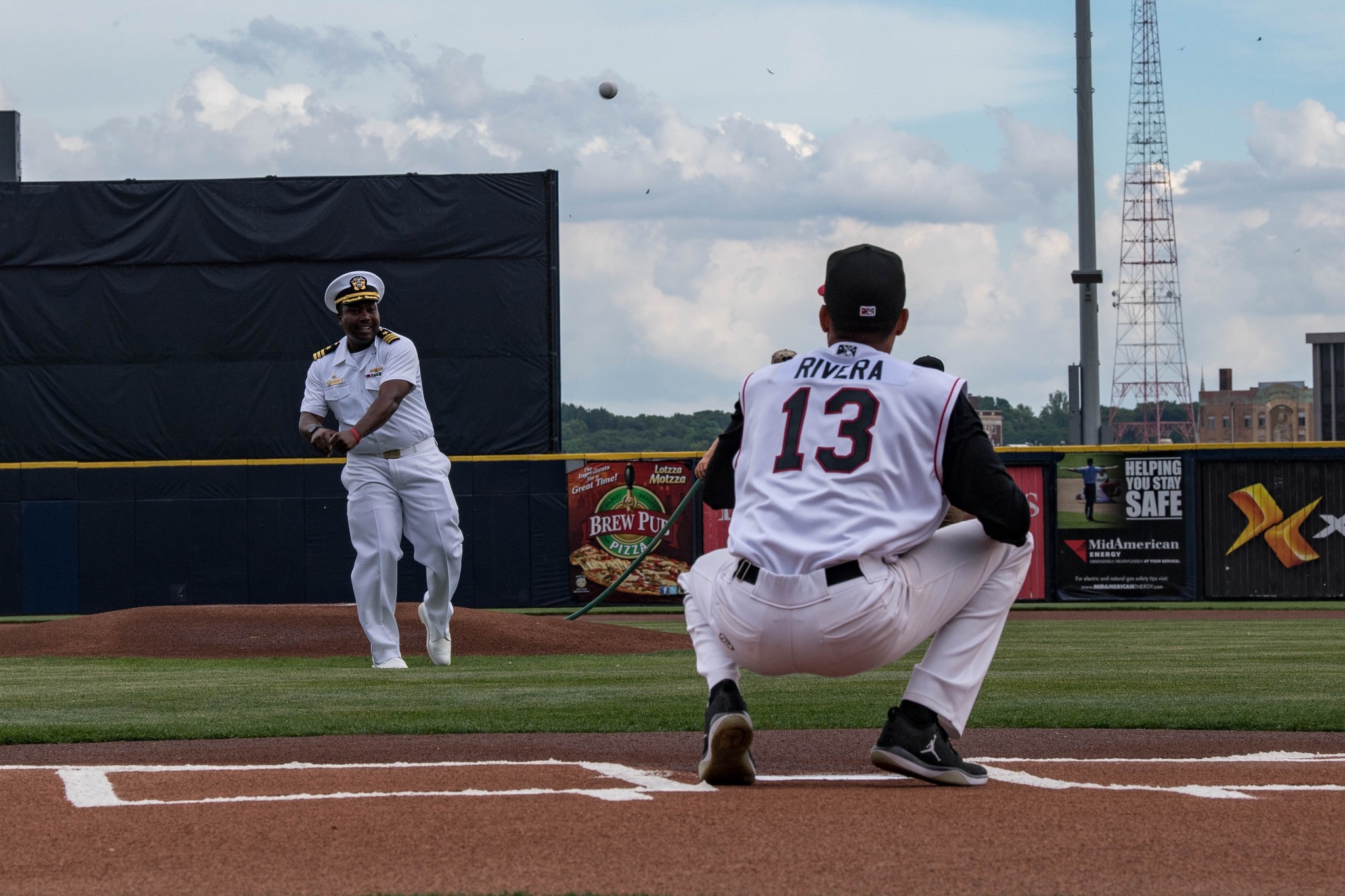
<box><xmin>772</xmin><ymin>349</ymin><xmax>798</xmax><ymax>365</ymax></box>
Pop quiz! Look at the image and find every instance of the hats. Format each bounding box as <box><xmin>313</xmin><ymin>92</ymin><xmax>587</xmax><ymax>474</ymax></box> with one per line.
<box><xmin>324</xmin><ymin>271</ymin><xmax>385</xmax><ymax>314</ymax></box>
<box><xmin>818</xmin><ymin>244</ymin><xmax>907</xmax><ymax>329</ymax></box>
<box><xmin>771</xmin><ymin>349</ymin><xmax>798</xmax><ymax>364</ymax></box>
<box><xmin>913</xmin><ymin>355</ymin><xmax>945</xmax><ymax>372</ymax></box>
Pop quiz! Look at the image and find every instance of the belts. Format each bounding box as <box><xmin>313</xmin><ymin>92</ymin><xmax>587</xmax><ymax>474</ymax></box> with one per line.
<box><xmin>367</xmin><ymin>436</ymin><xmax>436</xmax><ymax>458</ymax></box>
<box><xmin>735</xmin><ymin>559</ymin><xmax>861</xmax><ymax>592</ymax></box>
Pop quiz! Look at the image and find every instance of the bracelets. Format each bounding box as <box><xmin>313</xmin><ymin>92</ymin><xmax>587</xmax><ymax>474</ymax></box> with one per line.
<box><xmin>349</xmin><ymin>427</ymin><xmax>361</xmax><ymax>444</ymax></box>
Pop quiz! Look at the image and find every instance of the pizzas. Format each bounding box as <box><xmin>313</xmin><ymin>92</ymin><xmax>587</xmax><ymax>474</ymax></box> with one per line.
<box><xmin>570</xmin><ymin>545</ymin><xmax>694</xmax><ymax>595</ymax></box>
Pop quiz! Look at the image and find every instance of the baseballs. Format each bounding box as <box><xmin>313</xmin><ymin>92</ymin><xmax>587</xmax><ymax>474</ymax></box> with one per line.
<box><xmin>599</xmin><ymin>80</ymin><xmax>618</xmax><ymax>99</ymax></box>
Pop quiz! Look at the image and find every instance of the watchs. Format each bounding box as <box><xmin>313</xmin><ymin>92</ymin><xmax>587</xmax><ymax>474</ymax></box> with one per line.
<box><xmin>307</xmin><ymin>423</ymin><xmax>323</xmax><ymax>439</ymax></box>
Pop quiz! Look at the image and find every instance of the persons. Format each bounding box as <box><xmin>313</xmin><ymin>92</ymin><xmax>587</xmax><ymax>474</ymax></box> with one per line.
<box><xmin>299</xmin><ymin>271</ymin><xmax>464</xmax><ymax>669</ymax></box>
<box><xmin>1059</xmin><ymin>458</ymin><xmax>1125</xmax><ymax>522</ymax></box>
<box><xmin>694</xmin><ymin>349</ymin><xmax>797</xmax><ymax>480</ymax></box>
<box><xmin>912</xmin><ymin>354</ymin><xmax>945</xmax><ymax>373</ymax></box>
<box><xmin>677</xmin><ymin>243</ymin><xmax>1034</xmax><ymax>787</ymax></box>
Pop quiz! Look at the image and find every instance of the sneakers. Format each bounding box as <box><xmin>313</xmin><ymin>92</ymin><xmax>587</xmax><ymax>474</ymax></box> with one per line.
<box><xmin>870</xmin><ymin>706</ymin><xmax>988</xmax><ymax>785</ymax></box>
<box><xmin>371</xmin><ymin>658</ymin><xmax>408</xmax><ymax>669</ymax></box>
<box><xmin>418</xmin><ymin>602</ymin><xmax>452</xmax><ymax>664</ymax></box>
<box><xmin>698</xmin><ymin>679</ymin><xmax>756</xmax><ymax>786</ymax></box>
